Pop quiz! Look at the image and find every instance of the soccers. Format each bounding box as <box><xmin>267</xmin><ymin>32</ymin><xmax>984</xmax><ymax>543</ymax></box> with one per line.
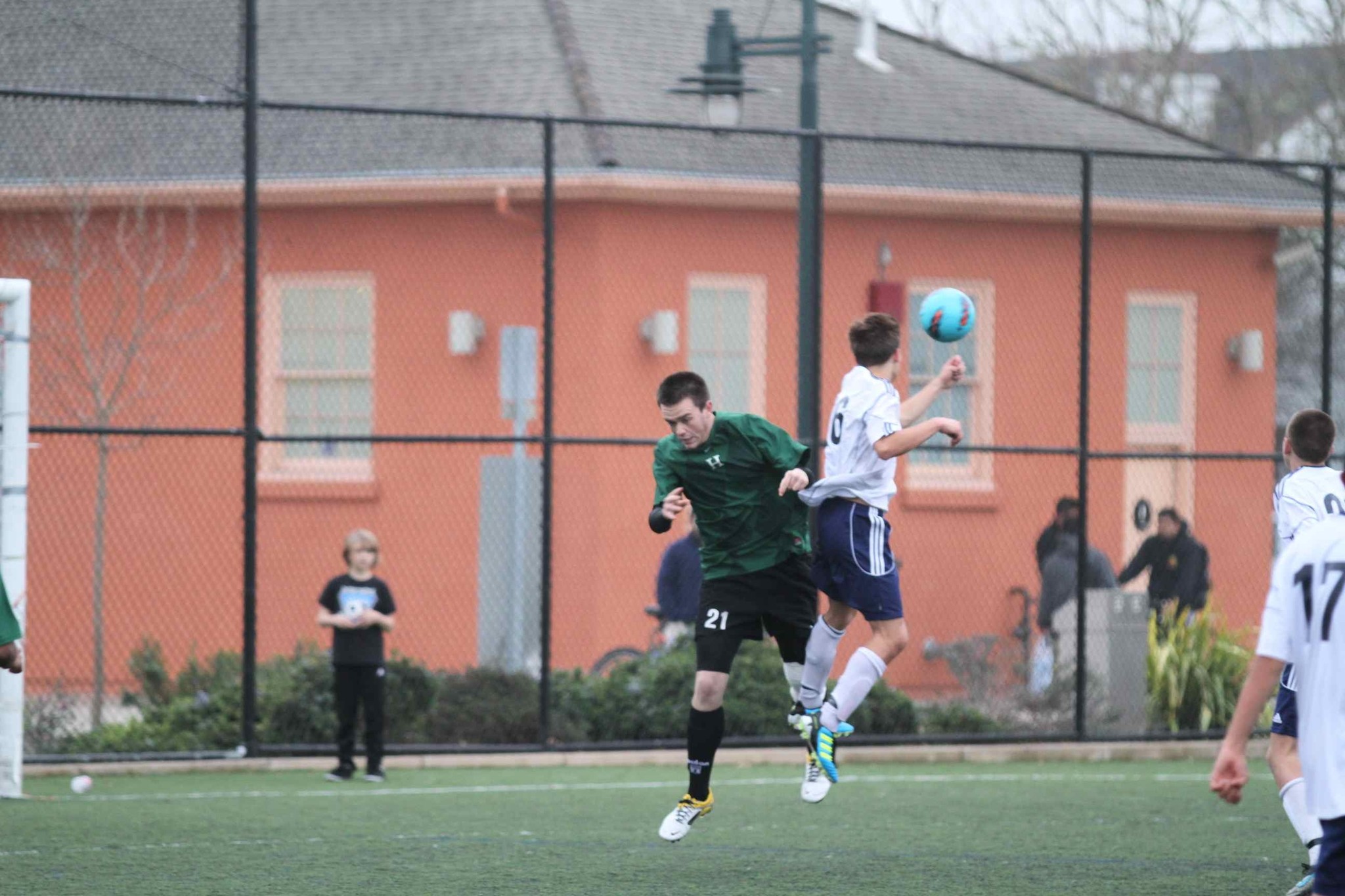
<box><xmin>919</xmin><ymin>288</ymin><xmax>975</xmax><ymax>342</ymax></box>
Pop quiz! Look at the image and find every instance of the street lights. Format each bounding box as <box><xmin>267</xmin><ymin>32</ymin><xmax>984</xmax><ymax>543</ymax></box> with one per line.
<box><xmin>664</xmin><ymin>7</ymin><xmax>840</xmax><ymax>625</ymax></box>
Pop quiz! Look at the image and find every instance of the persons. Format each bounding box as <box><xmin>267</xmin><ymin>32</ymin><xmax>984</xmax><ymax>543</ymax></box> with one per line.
<box><xmin>654</xmin><ymin>508</ymin><xmax>702</xmax><ymax>650</ymax></box>
<box><xmin>317</xmin><ymin>527</ymin><xmax>399</xmax><ymax>783</ymax></box>
<box><xmin>646</xmin><ymin>371</ymin><xmax>833</xmax><ymax>844</ymax></box>
<box><xmin>1034</xmin><ymin>517</ymin><xmax>1118</xmax><ymax>639</ymax></box>
<box><xmin>1035</xmin><ymin>495</ymin><xmax>1081</xmax><ymax>573</ymax></box>
<box><xmin>1209</xmin><ymin>405</ymin><xmax>1344</xmax><ymax>895</ymax></box>
<box><xmin>785</xmin><ymin>312</ymin><xmax>967</xmax><ymax>785</ymax></box>
<box><xmin>1118</xmin><ymin>508</ymin><xmax>1212</xmax><ymax>685</ymax></box>
<box><xmin>0</xmin><ymin>573</ymin><xmax>23</xmax><ymax>674</ymax></box>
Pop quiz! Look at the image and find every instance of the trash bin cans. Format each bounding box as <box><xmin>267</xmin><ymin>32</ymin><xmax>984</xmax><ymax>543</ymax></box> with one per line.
<box><xmin>1051</xmin><ymin>590</ymin><xmax>1152</xmax><ymax>732</ymax></box>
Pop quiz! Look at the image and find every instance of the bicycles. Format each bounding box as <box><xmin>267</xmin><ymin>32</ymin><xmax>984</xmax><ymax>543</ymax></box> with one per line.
<box><xmin>588</xmin><ymin>604</ymin><xmax>693</xmax><ymax>681</ymax></box>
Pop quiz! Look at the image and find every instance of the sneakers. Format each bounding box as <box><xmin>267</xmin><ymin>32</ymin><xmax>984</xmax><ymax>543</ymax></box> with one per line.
<box><xmin>323</xmin><ymin>768</ymin><xmax>386</xmax><ymax>785</ymax></box>
<box><xmin>787</xmin><ymin>700</ymin><xmax>854</xmax><ymax>803</ymax></box>
<box><xmin>658</xmin><ymin>788</ymin><xmax>714</xmax><ymax>842</ymax></box>
<box><xmin>1284</xmin><ymin>867</ymin><xmax>1317</xmax><ymax>896</ymax></box>
